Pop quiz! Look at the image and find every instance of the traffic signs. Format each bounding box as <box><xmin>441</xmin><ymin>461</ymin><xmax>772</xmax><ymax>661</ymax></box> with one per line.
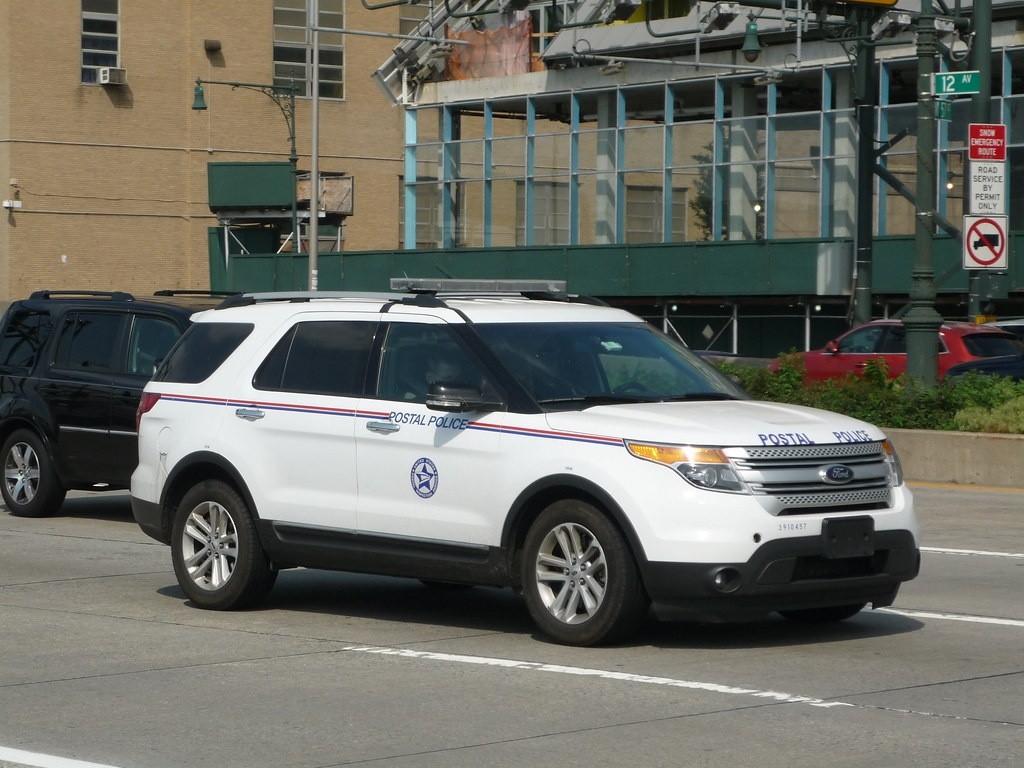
<box><xmin>931</xmin><ymin>71</ymin><xmax>983</xmax><ymax>94</ymax></box>
<box><xmin>933</xmin><ymin>97</ymin><xmax>954</xmax><ymax>122</ymax></box>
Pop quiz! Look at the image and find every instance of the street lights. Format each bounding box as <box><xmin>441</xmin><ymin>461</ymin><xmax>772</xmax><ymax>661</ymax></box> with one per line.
<box><xmin>739</xmin><ymin>9</ymin><xmax>871</xmax><ymax>344</ymax></box>
<box><xmin>192</xmin><ymin>78</ymin><xmax>300</xmax><ymax>256</ymax></box>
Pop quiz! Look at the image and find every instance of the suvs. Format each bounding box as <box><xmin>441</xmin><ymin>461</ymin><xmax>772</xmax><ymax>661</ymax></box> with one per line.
<box><xmin>128</xmin><ymin>277</ymin><xmax>924</xmax><ymax>648</ymax></box>
<box><xmin>1</xmin><ymin>289</ymin><xmax>252</xmax><ymax>516</ymax></box>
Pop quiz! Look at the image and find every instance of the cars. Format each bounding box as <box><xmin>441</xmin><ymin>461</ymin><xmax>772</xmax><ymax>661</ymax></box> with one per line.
<box><xmin>946</xmin><ymin>353</ymin><xmax>1024</xmax><ymax>389</ymax></box>
<box><xmin>768</xmin><ymin>318</ymin><xmax>1024</xmax><ymax>401</ymax></box>
